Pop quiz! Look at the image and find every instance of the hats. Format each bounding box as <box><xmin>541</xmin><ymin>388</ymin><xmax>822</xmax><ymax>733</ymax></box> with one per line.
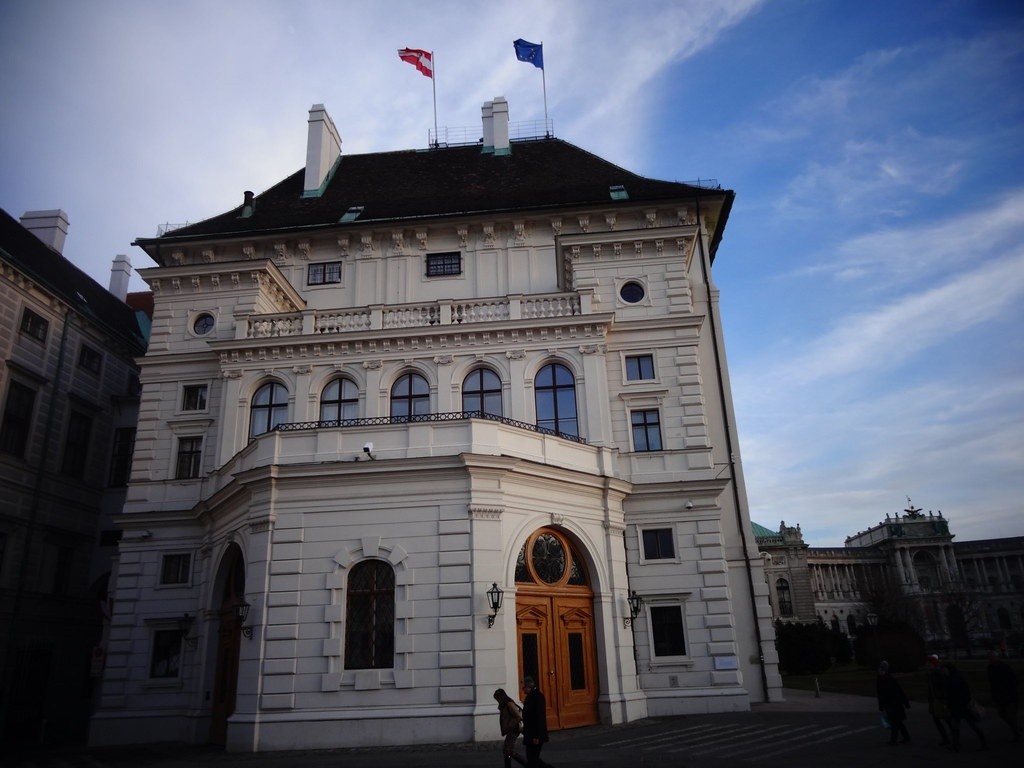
<box><xmin>521</xmin><ymin>676</ymin><xmax>536</xmax><ymax>688</ymax></box>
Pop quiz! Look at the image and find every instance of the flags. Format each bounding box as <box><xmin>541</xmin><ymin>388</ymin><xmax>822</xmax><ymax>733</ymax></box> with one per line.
<box><xmin>398</xmin><ymin>48</ymin><xmax>432</xmax><ymax>79</ymax></box>
<box><xmin>514</xmin><ymin>39</ymin><xmax>543</xmax><ymax>69</ymax></box>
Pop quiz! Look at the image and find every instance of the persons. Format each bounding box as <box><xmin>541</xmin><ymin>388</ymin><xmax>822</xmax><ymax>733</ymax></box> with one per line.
<box><xmin>979</xmin><ymin>653</ymin><xmax>1024</xmax><ymax>742</ymax></box>
<box><xmin>521</xmin><ymin>677</ymin><xmax>555</xmax><ymax>768</ymax></box>
<box><xmin>877</xmin><ymin>661</ymin><xmax>912</xmax><ymax>746</ymax></box>
<box><xmin>922</xmin><ymin>653</ymin><xmax>959</xmax><ymax>749</ymax></box>
<box><xmin>940</xmin><ymin>659</ymin><xmax>990</xmax><ymax>750</ymax></box>
<box><xmin>494</xmin><ymin>689</ymin><xmax>528</xmax><ymax>768</ymax></box>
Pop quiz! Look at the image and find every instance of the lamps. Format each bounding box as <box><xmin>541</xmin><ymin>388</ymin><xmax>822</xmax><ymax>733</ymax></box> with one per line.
<box><xmin>623</xmin><ymin>590</ymin><xmax>641</xmax><ymax>629</ymax></box>
<box><xmin>486</xmin><ymin>582</ymin><xmax>504</xmax><ymax>628</ymax></box>
<box><xmin>177</xmin><ymin>613</ymin><xmax>198</xmax><ymax>650</ymax></box>
<box><xmin>232</xmin><ymin>596</ymin><xmax>253</xmax><ymax>640</ymax></box>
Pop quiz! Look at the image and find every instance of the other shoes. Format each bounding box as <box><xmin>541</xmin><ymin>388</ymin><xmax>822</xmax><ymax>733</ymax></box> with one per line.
<box><xmin>976</xmin><ymin>745</ymin><xmax>990</xmax><ymax>751</ymax></box>
<box><xmin>947</xmin><ymin>746</ymin><xmax>960</xmax><ymax>752</ymax></box>
<box><xmin>902</xmin><ymin>739</ymin><xmax>912</xmax><ymax>745</ymax></box>
<box><xmin>887</xmin><ymin>738</ymin><xmax>898</xmax><ymax>744</ymax></box>
<box><xmin>937</xmin><ymin>740</ymin><xmax>948</xmax><ymax>747</ymax></box>
